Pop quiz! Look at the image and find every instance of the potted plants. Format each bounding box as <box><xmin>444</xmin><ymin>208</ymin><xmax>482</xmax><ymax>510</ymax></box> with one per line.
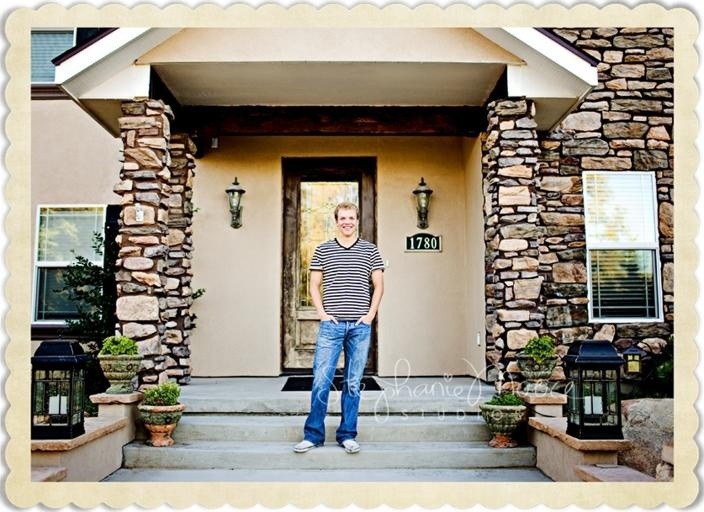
<box><xmin>515</xmin><ymin>335</ymin><xmax>559</xmax><ymax>393</ymax></box>
<box><xmin>479</xmin><ymin>391</ymin><xmax>527</xmax><ymax>447</ymax></box>
<box><xmin>97</xmin><ymin>336</ymin><xmax>144</xmax><ymax>394</ymax></box>
<box><xmin>137</xmin><ymin>381</ymin><xmax>186</xmax><ymax>447</ymax></box>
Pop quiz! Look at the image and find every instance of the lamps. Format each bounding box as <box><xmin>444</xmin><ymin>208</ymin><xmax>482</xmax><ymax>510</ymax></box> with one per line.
<box><xmin>413</xmin><ymin>178</ymin><xmax>433</xmax><ymax>228</ymax></box>
<box><xmin>225</xmin><ymin>177</ymin><xmax>246</xmax><ymax>228</ymax></box>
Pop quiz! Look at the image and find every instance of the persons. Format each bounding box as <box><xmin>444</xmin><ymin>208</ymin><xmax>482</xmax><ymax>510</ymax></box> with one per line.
<box><xmin>293</xmin><ymin>201</ymin><xmax>385</xmax><ymax>454</ymax></box>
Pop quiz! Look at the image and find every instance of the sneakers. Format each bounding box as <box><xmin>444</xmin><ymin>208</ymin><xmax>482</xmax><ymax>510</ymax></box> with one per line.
<box><xmin>342</xmin><ymin>439</ymin><xmax>360</xmax><ymax>454</ymax></box>
<box><xmin>294</xmin><ymin>439</ymin><xmax>317</xmax><ymax>452</ymax></box>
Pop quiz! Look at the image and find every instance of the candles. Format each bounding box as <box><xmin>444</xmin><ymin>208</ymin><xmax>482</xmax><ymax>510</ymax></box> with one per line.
<box><xmin>49</xmin><ymin>394</ymin><xmax>67</xmax><ymax>415</ymax></box>
<box><xmin>585</xmin><ymin>396</ymin><xmax>603</xmax><ymax>415</ymax></box>
